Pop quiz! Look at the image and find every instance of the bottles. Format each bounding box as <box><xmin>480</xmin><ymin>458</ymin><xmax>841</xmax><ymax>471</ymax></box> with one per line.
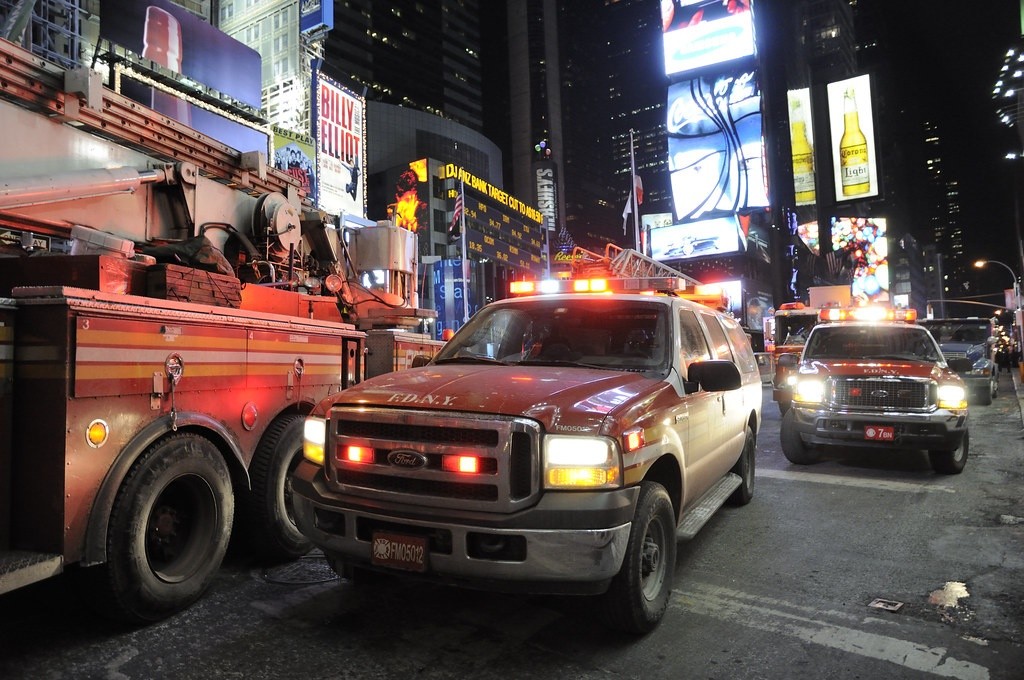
<box><xmin>790</xmin><ymin>99</ymin><xmax>816</xmax><ymax>206</ymax></box>
<box><xmin>840</xmin><ymin>89</ymin><xmax>870</xmax><ymax>195</ymax></box>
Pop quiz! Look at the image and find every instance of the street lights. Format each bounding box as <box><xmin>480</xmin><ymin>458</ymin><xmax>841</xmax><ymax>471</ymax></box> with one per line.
<box><xmin>974</xmin><ymin>260</ymin><xmax>1024</xmax><ymax>383</ymax></box>
<box><xmin>994</xmin><ymin>309</ymin><xmax>1013</xmax><ymax>317</ymax></box>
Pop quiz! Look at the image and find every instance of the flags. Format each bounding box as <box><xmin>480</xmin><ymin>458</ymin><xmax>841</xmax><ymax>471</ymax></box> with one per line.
<box><xmin>448</xmin><ymin>177</ymin><xmax>462</xmax><ymax>232</ymax></box>
<box><xmin>622</xmin><ymin>161</ymin><xmax>643</xmax><ymax>235</ymax></box>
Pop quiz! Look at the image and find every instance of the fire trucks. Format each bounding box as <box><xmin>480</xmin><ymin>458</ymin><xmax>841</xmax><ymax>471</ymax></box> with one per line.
<box><xmin>289</xmin><ymin>244</ymin><xmax>762</xmax><ymax>625</ymax></box>
<box><xmin>772</xmin><ymin>285</ymin><xmax>970</xmax><ymax>474</ymax></box>
<box><xmin>0</xmin><ymin>36</ymin><xmax>455</xmax><ymax>602</ymax></box>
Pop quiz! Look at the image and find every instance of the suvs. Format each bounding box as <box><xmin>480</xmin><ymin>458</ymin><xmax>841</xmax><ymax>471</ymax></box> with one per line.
<box><xmin>917</xmin><ymin>317</ymin><xmax>999</xmax><ymax>405</ymax></box>
